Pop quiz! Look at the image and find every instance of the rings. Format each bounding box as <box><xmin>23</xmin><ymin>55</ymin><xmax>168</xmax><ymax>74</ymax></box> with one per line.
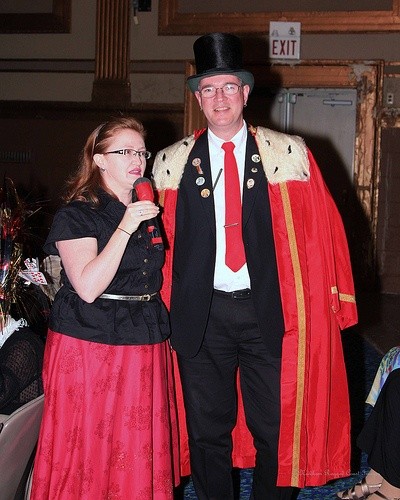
<box><xmin>139</xmin><ymin>210</ymin><xmax>143</xmax><ymax>216</ymax></box>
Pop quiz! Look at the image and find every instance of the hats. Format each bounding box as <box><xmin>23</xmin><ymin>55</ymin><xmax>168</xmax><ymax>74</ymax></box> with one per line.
<box><xmin>186</xmin><ymin>33</ymin><xmax>255</xmax><ymax>95</ymax></box>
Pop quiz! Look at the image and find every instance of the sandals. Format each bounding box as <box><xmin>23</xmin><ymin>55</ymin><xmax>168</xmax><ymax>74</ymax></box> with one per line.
<box><xmin>336</xmin><ymin>477</ymin><xmax>391</xmax><ymax>500</ymax></box>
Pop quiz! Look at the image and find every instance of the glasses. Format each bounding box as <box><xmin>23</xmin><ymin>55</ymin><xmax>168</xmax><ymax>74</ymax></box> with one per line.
<box><xmin>101</xmin><ymin>149</ymin><xmax>151</xmax><ymax>160</ymax></box>
<box><xmin>199</xmin><ymin>84</ymin><xmax>243</xmax><ymax>98</ymax></box>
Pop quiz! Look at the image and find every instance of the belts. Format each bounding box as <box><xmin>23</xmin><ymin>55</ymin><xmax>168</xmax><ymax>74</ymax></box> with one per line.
<box><xmin>69</xmin><ymin>288</ymin><xmax>156</xmax><ymax>303</ymax></box>
<box><xmin>212</xmin><ymin>288</ymin><xmax>253</xmax><ymax>299</ymax></box>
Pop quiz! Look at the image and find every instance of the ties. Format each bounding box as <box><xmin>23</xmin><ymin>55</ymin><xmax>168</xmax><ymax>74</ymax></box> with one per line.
<box><xmin>221</xmin><ymin>142</ymin><xmax>247</xmax><ymax>273</ymax></box>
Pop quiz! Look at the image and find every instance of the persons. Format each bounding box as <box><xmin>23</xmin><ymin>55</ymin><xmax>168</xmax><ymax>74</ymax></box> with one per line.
<box><xmin>0</xmin><ymin>178</ymin><xmax>43</xmax><ymax>424</ymax></box>
<box><xmin>337</xmin><ymin>345</ymin><xmax>400</xmax><ymax>500</ymax></box>
<box><xmin>151</xmin><ymin>32</ymin><xmax>358</xmax><ymax>500</ymax></box>
<box><xmin>30</xmin><ymin>116</ymin><xmax>191</xmax><ymax>500</ymax></box>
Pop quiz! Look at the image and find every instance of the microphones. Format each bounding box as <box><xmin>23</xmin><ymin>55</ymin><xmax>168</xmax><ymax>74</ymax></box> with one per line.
<box><xmin>132</xmin><ymin>178</ymin><xmax>163</xmax><ymax>251</ymax></box>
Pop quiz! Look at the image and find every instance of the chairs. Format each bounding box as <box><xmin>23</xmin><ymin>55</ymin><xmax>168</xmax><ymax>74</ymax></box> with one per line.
<box><xmin>0</xmin><ymin>394</ymin><xmax>44</xmax><ymax>500</ymax></box>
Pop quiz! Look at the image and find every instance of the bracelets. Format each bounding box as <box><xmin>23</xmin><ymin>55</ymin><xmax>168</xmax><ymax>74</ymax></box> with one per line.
<box><xmin>117</xmin><ymin>228</ymin><xmax>131</xmax><ymax>236</ymax></box>
